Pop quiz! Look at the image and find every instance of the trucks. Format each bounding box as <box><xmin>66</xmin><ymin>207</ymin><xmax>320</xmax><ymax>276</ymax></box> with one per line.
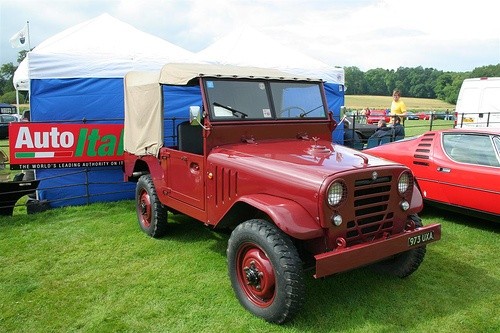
<box><xmin>0</xmin><ymin>103</ymin><xmax>19</xmax><ymax>140</ymax></box>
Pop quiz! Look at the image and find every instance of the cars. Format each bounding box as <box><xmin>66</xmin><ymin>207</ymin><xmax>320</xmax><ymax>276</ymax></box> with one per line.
<box><xmin>367</xmin><ymin>110</ymin><xmax>455</xmax><ymax>127</ymax></box>
<box><xmin>361</xmin><ymin>130</ymin><xmax>500</xmax><ymax>220</ymax></box>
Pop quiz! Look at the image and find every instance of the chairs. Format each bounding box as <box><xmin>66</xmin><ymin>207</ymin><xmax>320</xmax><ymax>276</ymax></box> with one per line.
<box><xmin>177</xmin><ymin>117</ymin><xmax>204</xmax><ymax>156</ymax></box>
<box><xmin>344</xmin><ymin>125</ymin><xmax>405</xmax><ymax>150</ymax></box>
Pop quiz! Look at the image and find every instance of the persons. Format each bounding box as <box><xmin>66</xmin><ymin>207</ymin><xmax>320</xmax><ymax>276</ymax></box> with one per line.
<box><xmin>374</xmin><ymin>120</ymin><xmax>390</xmax><ymax>135</ymax></box>
<box><xmin>343</xmin><ymin>118</ymin><xmax>363</xmax><ymax>150</ymax></box>
<box><xmin>389</xmin><ymin>89</ymin><xmax>407</xmax><ymax>125</ymax></box>
<box><xmin>389</xmin><ymin>116</ymin><xmax>405</xmax><ymax>142</ymax></box>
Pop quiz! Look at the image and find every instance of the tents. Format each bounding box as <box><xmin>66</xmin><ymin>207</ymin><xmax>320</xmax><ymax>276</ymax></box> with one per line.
<box><xmin>12</xmin><ymin>10</ymin><xmax>344</xmax><ymax>209</ymax></box>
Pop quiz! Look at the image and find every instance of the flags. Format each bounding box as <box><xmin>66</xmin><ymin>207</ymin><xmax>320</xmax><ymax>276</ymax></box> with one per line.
<box><xmin>9</xmin><ymin>24</ymin><xmax>29</xmax><ymax>48</ymax></box>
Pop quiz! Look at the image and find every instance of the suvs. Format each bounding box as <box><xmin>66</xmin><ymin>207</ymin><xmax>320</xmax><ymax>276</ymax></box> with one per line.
<box><xmin>122</xmin><ymin>75</ymin><xmax>442</xmax><ymax>325</ymax></box>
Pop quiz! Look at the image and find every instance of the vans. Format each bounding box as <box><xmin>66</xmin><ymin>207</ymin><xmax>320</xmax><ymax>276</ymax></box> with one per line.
<box><xmin>453</xmin><ymin>77</ymin><xmax>500</xmax><ymax>130</ymax></box>
